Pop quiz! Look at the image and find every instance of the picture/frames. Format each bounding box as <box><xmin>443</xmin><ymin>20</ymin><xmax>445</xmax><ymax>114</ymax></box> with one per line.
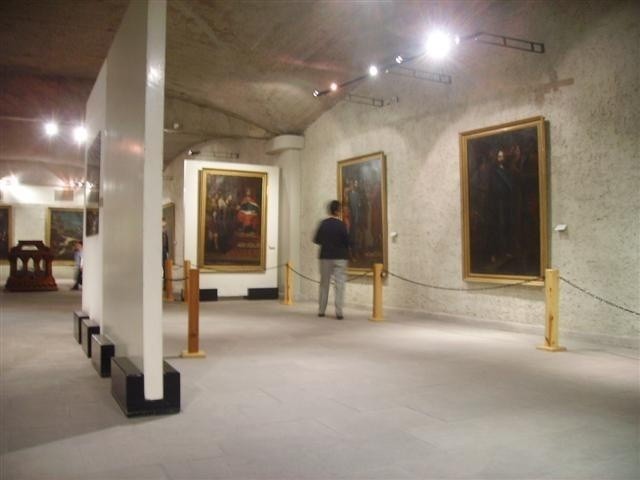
<box><xmin>457</xmin><ymin>114</ymin><xmax>552</xmax><ymax>289</ymax></box>
<box><xmin>43</xmin><ymin>206</ymin><xmax>85</xmax><ymax>268</ymax></box>
<box><xmin>333</xmin><ymin>149</ymin><xmax>391</xmax><ymax>279</ymax></box>
<box><xmin>162</xmin><ymin>201</ymin><xmax>176</xmax><ymax>268</ymax></box>
<box><xmin>0</xmin><ymin>204</ymin><xmax>18</xmax><ymax>267</ymax></box>
<box><xmin>193</xmin><ymin>166</ymin><xmax>270</xmax><ymax>276</ymax></box>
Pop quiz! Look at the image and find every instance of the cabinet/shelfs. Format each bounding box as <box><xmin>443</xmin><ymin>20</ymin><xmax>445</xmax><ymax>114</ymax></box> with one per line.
<box><xmin>3</xmin><ymin>239</ymin><xmax>58</xmax><ymax>292</ymax></box>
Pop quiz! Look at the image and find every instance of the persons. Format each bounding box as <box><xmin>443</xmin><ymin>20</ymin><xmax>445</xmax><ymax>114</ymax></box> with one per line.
<box><xmin>474</xmin><ymin>144</ymin><xmax>535</xmax><ymax>262</ymax></box>
<box><xmin>314</xmin><ymin>201</ymin><xmax>352</xmax><ymax>320</ymax></box>
<box><xmin>163</xmin><ymin>221</ymin><xmax>168</xmax><ymax>290</ymax></box>
<box><xmin>70</xmin><ymin>240</ymin><xmax>83</xmax><ymax>290</ymax></box>
